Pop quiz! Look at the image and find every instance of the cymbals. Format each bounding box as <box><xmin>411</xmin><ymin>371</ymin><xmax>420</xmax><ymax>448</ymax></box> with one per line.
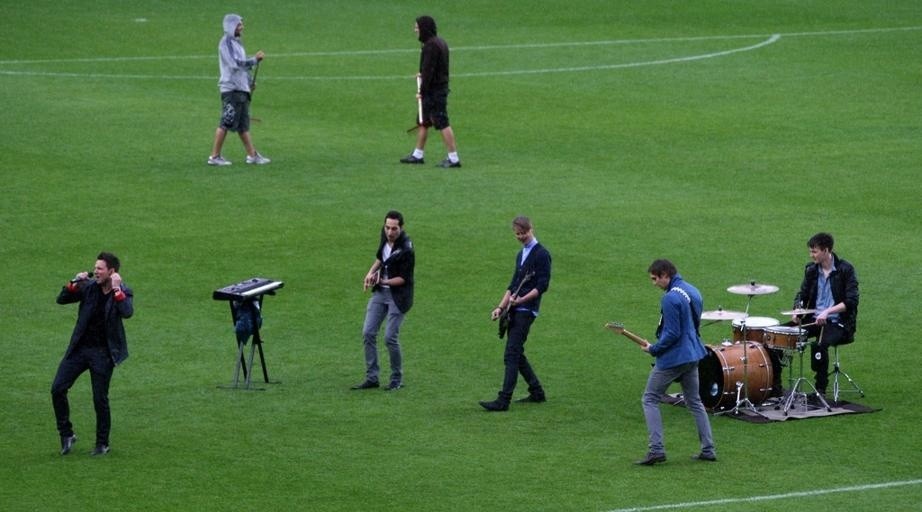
<box><xmin>727</xmin><ymin>284</ymin><xmax>779</xmax><ymax>296</ymax></box>
<box><xmin>698</xmin><ymin>309</ymin><xmax>748</xmax><ymax>324</ymax></box>
<box><xmin>782</xmin><ymin>309</ymin><xmax>814</xmax><ymax>316</ymax></box>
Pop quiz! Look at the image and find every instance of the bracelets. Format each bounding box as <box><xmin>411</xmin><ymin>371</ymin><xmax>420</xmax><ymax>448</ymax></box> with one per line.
<box><xmin>496</xmin><ymin>306</ymin><xmax>506</xmax><ymax>315</ymax></box>
<box><xmin>112</xmin><ymin>287</ymin><xmax>121</xmax><ymax>295</ymax></box>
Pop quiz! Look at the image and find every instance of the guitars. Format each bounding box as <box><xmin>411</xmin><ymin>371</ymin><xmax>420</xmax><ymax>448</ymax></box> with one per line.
<box><xmin>604</xmin><ymin>318</ymin><xmax>648</xmax><ymax>349</ymax></box>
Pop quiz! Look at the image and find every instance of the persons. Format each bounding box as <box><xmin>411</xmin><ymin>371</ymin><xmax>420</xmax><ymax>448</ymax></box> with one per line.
<box><xmin>634</xmin><ymin>255</ymin><xmax>719</xmax><ymax>467</ymax></box>
<box><xmin>51</xmin><ymin>251</ymin><xmax>136</xmax><ymax>459</ymax></box>
<box><xmin>350</xmin><ymin>206</ymin><xmax>416</xmax><ymax>392</ymax></box>
<box><xmin>479</xmin><ymin>213</ymin><xmax>553</xmax><ymax>411</ymax></box>
<box><xmin>399</xmin><ymin>14</ymin><xmax>464</xmax><ymax>169</ymax></box>
<box><xmin>204</xmin><ymin>12</ymin><xmax>272</xmax><ymax>168</ymax></box>
<box><xmin>762</xmin><ymin>231</ymin><xmax>860</xmax><ymax>404</ymax></box>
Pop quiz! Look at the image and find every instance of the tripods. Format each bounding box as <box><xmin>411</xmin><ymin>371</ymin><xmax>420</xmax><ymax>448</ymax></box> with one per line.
<box><xmin>729</xmin><ymin>323</ymin><xmax>758</xmax><ymax>414</ymax></box>
<box><xmin>774</xmin><ymin>352</ymin><xmax>806</xmax><ymax>410</ymax></box>
<box><xmin>783</xmin><ymin>338</ymin><xmax>832</xmax><ymax>416</ymax></box>
<box><xmin>827</xmin><ymin>346</ymin><xmax>866</xmax><ymax>407</ymax></box>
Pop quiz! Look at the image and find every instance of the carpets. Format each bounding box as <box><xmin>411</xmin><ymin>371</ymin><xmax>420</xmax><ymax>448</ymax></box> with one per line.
<box><xmin>662</xmin><ymin>391</ymin><xmax>882</xmax><ymax>424</ymax></box>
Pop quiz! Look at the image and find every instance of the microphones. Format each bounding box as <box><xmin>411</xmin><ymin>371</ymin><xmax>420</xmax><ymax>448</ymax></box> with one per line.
<box><xmin>70</xmin><ymin>272</ymin><xmax>94</xmax><ymax>284</ymax></box>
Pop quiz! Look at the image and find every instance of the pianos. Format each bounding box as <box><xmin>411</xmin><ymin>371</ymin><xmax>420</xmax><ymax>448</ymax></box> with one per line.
<box><xmin>209</xmin><ymin>276</ymin><xmax>284</xmax><ymax>389</ymax></box>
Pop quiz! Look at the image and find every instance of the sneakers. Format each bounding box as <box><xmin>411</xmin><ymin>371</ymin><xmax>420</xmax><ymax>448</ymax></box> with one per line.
<box><xmin>802</xmin><ymin>392</ymin><xmax>825</xmax><ymax>406</ymax></box>
<box><xmin>208</xmin><ymin>155</ymin><xmax>233</xmax><ymax>166</ymax></box>
<box><xmin>766</xmin><ymin>385</ymin><xmax>785</xmax><ymax>402</ymax></box>
<box><xmin>400</xmin><ymin>152</ymin><xmax>423</xmax><ymax>164</ymax></box>
<box><xmin>246</xmin><ymin>151</ymin><xmax>271</xmax><ymax>165</ymax></box>
<box><xmin>437</xmin><ymin>158</ymin><xmax>461</xmax><ymax>168</ymax></box>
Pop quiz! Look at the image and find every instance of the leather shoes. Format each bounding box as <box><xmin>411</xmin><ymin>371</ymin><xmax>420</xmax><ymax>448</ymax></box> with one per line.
<box><xmin>60</xmin><ymin>434</ymin><xmax>77</xmax><ymax>455</ymax></box>
<box><xmin>692</xmin><ymin>452</ymin><xmax>715</xmax><ymax>461</ymax></box>
<box><xmin>384</xmin><ymin>382</ymin><xmax>403</xmax><ymax>392</ymax></box>
<box><xmin>515</xmin><ymin>393</ymin><xmax>546</xmax><ymax>403</ymax></box>
<box><xmin>350</xmin><ymin>381</ymin><xmax>379</xmax><ymax>389</ymax></box>
<box><xmin>91</xmin><ymin>443</ymin><xmax>110</xmax><ymax>455</ymax></box>
<box><xmin>479</xmin><ymin>400</ymin><xmax>509</xmax><ymax>410</ymax></box>
<box><xmin>633</xmin><ymin>453</ymin><xmax>665</xmax><ymax>465</ymax></box>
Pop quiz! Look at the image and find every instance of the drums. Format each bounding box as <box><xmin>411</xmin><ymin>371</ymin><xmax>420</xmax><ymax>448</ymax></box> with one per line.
<box><xmin>731</xmin><ymin>316</ymin><xmax>779</xmax><ymax>344</ymax></box>
<box><xmin>762</xmin><ymin>324</ymin><xmax>806</xmax><ymax>350</ymax></box>
<box><xmin>699</xmin><ymin>339</ymin><xmax>774</xmax><ymax>412</ymax></box>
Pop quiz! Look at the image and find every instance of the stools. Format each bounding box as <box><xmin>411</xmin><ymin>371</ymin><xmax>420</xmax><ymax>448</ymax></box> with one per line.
<box><xmin>829</xmin><ymin>334</ymin><xmax>866</xmax><ymax>407</ymax></box>
